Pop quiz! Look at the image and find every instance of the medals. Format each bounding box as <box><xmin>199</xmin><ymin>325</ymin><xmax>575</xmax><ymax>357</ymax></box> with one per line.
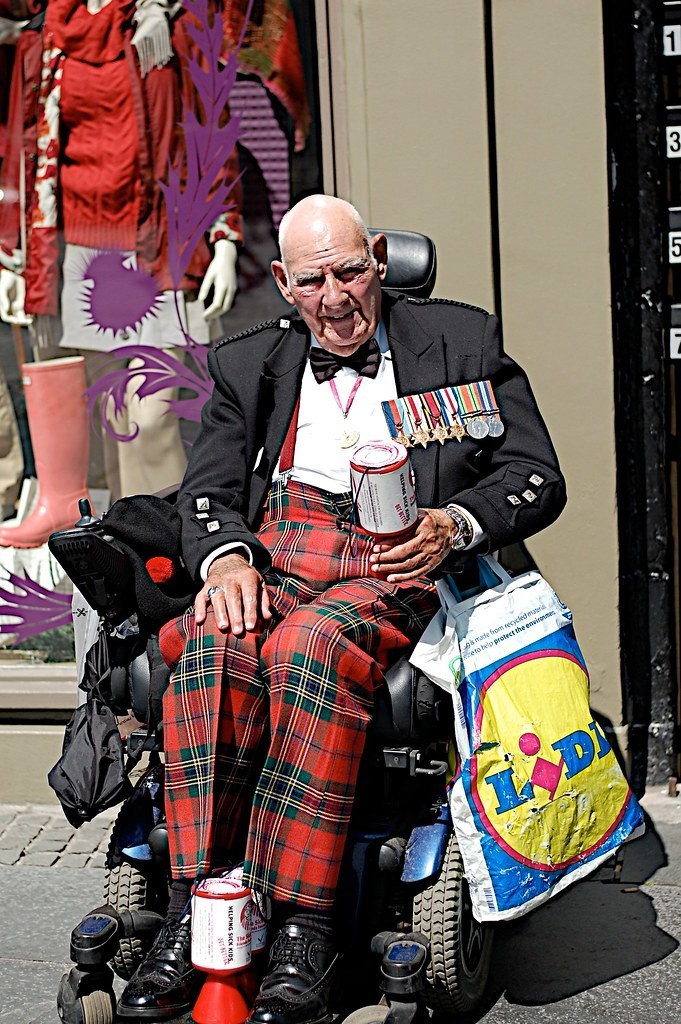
<box><xmin>335</xmin><ymin>421</ymin><xmax>360</xmax><ymax>450</ymax></box>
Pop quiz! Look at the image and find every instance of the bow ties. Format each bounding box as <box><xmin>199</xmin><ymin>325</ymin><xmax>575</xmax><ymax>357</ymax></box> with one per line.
<box><xmin>310</xmin><ymin>338</ymin><xmax>381</xmax><ymax>384</ymax></box>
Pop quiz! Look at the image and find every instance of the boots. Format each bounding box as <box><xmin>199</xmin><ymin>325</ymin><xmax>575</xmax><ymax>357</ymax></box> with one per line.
<box><xmin>0</xmin><ymin>357</ymin><xmax>96</xmax><ymax>548</ymax></box>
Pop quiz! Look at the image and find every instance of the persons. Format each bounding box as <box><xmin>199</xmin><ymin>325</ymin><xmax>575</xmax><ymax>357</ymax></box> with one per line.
<box><xmin>0</xmin><ymin>0</ymin><xmax>310</xmax><ymax>499</ymax></box>
<box><xmin>108</xmin><ymin>192</ymin><xmax>570</xmax><ymax>1023</ymax></box>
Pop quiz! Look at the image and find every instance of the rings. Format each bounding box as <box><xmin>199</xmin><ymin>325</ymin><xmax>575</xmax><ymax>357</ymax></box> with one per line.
<box><xmin>207</xmin><ymin>584</ymin><xmax>225</xmax><ymax>599</ymax></box>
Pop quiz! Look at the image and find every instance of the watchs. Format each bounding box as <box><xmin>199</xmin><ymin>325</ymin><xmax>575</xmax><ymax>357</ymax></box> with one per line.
<box><xmin>442</xmin><ymin>507</ymin><xmax>473</xmax><ymax>551</ymax></box>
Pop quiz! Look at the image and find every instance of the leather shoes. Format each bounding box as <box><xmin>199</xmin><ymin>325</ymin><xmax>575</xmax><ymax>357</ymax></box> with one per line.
<box><xmin>244</xmin><ymin>923</ymin><xmax>345</xmax><ymax>1024</ymax></box>
<box><xmin>117</xmin><ymin>918</ymin><xmax>208</xmax><ymax>1017</ymax></box>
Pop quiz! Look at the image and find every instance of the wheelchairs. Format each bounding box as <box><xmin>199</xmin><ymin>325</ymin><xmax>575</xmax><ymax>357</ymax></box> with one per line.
<box><xmin>51</xmin><ymin>226</ymin><xmax>502</xmax><ymax>1023</ymax></box>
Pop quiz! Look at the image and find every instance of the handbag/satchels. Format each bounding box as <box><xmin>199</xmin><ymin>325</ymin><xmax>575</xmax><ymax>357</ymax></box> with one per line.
<box><xmin>408</xmin><ymin>553</ymin><xmax>646</xmax><ymax>923</ymax></box>
